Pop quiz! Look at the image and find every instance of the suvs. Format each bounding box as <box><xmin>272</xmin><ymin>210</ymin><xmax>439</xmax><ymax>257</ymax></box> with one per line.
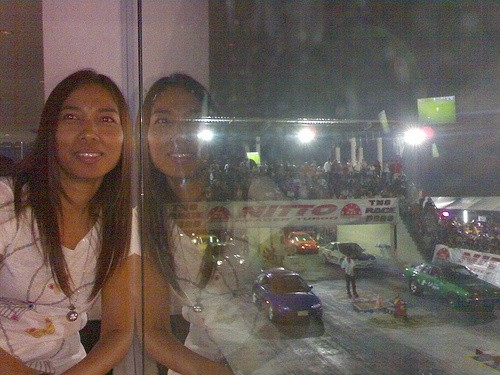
<box><xmin>204</xmin><ymin>242</ymin><xmax>247</xmax><ymax>274</ymax></box>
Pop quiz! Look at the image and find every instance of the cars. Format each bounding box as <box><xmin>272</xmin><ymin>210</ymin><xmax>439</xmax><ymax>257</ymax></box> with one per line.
<box><xmin>318</xmin><ymin>241</ymin><xmax>377</xmax><ymax>271</ymax></box>
<box><xmin>448</xmin><ymin>219</ymin><xmax>500</xmax><ymax>252</ymax></box>
<box><xmin>402</xmin><ymin>260</ymin><xmax>500</xmax><ymax>312</ymax></box>
<box><xmin>190</xmin><ymin>233</ymin><xmax>221</xmax><ymax>252</ymax></box>
<box><xmin>283</xmin><ymin>231</ymin><xmax>320</xmax><ymax>255</ymax></box>
<box><xmin>252</xmin><ymin>267</ymin><xmax>324</xmax><ymax>326</ymax></box>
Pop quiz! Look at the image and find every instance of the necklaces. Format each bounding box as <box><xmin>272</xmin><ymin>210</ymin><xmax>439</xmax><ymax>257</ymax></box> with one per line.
<box><xmin>67</xmin><ymin>209</ymin><xmax>93</xmax><ymax>321</ymax></box>
<box><xmin>173</xmin><ymin>199</ymin><xmax>204</xmax><ymax>313</ymax></box>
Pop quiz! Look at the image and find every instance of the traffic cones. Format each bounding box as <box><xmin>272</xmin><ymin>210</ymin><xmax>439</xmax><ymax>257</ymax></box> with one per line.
<box><xmin>374</xmin><ymin>294</ymin><xmax>383</xmax><ymax>311</ymax></box>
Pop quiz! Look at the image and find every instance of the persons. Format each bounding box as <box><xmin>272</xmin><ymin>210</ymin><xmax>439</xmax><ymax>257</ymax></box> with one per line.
<box><xmin>341</xmin><ymin>253</ymin><xmax>359</xmax><ymax>298</ymax></box>
<box><xmin>198</xmin><ymin>156</ymin><xmax>500</xmax><ymax>269</ymax></box>
<box><xmin>131</xmin><ymin>70</ymin><xmax>296</xmax><ymax>374</ymax></box>
<box><xmin>0</xmin><ymin>68</ymin><xmax>137</xmax><ymax>375</ymax></box>
